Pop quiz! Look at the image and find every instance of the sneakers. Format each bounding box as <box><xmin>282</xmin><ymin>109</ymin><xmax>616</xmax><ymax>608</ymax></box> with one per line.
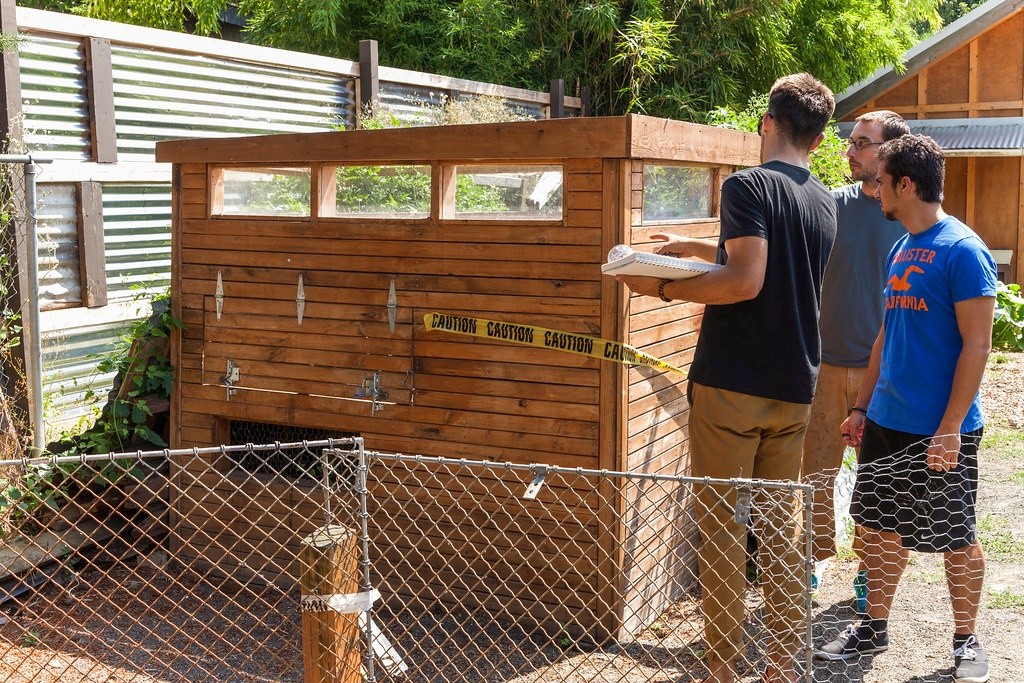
<box><xmin>815</xmin><ymin>615</ymin><xmax>888</xmax><ymax>660</ymax></box>
<box><xmin>953</xmin><ymin>635</ymin><xmax>990</xmax><ymax>683</ymax></box>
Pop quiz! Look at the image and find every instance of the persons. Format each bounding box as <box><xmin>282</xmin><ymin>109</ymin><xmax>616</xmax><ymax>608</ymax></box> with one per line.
<box><xmin>818</xmin><ymin>132</ymin><xmax>999</xmax><ymax>683</ymax></box>
<box><xmin>800</xmin><ymin>110</ymin><xmax>909</xmax><ymax>614</ymax></box>
<box><xmin>615</xmin><ymin>72</ymin><xmax>839</xmax><ymax>683</ymax></box>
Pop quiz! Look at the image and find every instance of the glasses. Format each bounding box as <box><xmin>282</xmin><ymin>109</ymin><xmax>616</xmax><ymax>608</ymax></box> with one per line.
<box><xmin>848</xmin><ymin>139</ymin><xmax>884</xmax><ymax>151</ymax></box>
<box><xmin>757</xmin><ymin>111</ymin><xmax>774</xmax><ymax>136</ymax></box>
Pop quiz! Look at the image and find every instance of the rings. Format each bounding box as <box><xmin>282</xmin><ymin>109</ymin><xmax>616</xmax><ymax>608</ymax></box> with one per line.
<box><xmin>841</xmin><ymin>433</ymin><xmax>850</xmax><ymax>438</ymax></box>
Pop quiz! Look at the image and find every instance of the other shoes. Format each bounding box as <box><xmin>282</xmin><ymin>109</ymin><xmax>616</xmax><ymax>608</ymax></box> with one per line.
<box><xmin>812</xmin><ymin>574</ymin><xmax>818</xmax><ymax>589</ymax></box>
<box><xmin>853</xmin><ymin>570</ymin><xmax>868</xmax><ymax>612</ymax></box>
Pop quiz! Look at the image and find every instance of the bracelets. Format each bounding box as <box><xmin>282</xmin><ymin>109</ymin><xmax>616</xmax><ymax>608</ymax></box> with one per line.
<box><xmin>658</xmin><ymin>279</ymin><xmax>672</xmax><ymax>302</ymax></box>
<box><xmin>851</xmin><ymin>407</ymin><xmax>866</xmax><ymax>413</ymax></box>
